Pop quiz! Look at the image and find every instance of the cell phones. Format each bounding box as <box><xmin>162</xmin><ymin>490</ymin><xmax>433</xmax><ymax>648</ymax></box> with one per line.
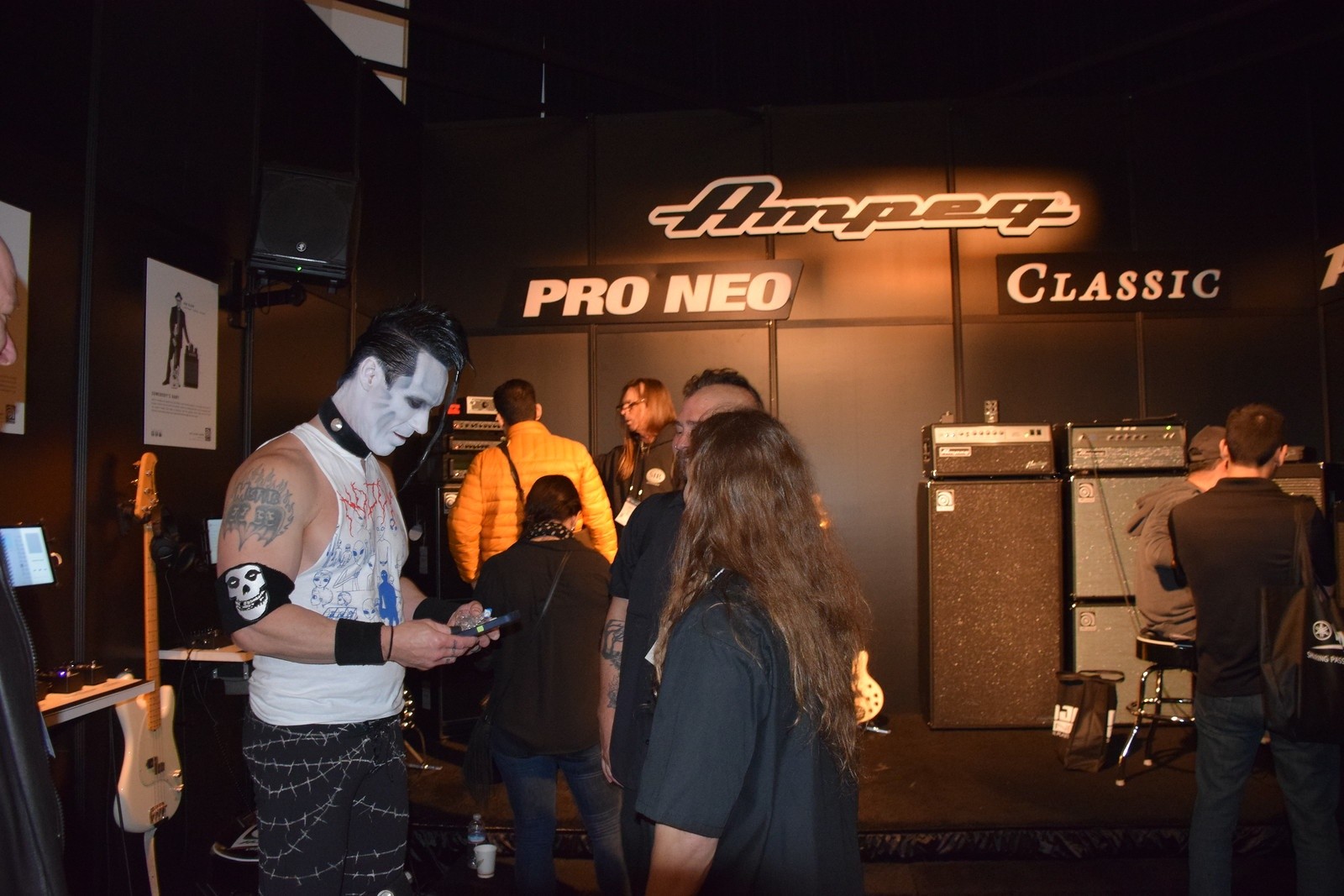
<box><xmin>456</xmin><ymin>608</ymin><xmax>520</xmax><ymax>637</ymax></box>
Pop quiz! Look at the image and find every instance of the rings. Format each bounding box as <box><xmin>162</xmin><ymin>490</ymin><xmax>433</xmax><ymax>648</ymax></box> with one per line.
<box><xmin>445</xmin><ymin>658</ymin><xmax>450</xmax><ymax>664</ymax></box>
<box><xmin>451</xmin><ymin>648</ymin><xmax>455</xmax><ymax>656</ymax></box>
<box><xmin>471</xmin><ymin>644</ymin><xmax>481</xmax><ymax>652</ymax></box>
<box><xmin>453</xmin><ymin>639</ymin><xmax>457</xmax><ymax>648</ymax></box>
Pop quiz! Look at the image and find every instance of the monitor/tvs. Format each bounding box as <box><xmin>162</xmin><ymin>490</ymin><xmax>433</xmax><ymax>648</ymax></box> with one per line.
<box><xmin>201</xmin><ymin>517</ymin><xmax>222</xmax><ymax>566</ymax></box>
<box><xmin>0</xmin><ymin>524</ymin><xmax>56</xmax><ymax>588</ymax></box>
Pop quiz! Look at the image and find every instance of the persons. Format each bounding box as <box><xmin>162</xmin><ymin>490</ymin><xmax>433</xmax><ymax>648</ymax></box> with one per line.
<box><xmin>162</xmin><ymin>292</ymin><xmax>190</xmax><ymax>385</ymax></box>
<box><xmin>1126</xmin><ymin>403</ymin><xmax>1343</xmax><ymax>896</ymax></box>
<box><xmin>447</xmin><ymin>368</ymin><xmax>871</xmax><ymax>896</ymax></box>
<box><xmin>0</xmin><ymin>234</ymin><xmax>62</xmax><ymax>896</ymax></box>
<box><xmin>217</xmin><ymin>303</ymin><xmax>500</xmax><ymax>895</ymax></box>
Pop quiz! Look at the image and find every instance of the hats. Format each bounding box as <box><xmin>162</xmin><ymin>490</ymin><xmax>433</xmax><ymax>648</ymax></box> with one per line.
<box><xmin>1186</xmin><ymin>424</ymin><xmax>1227</xmax><ymax>463</ymax></box>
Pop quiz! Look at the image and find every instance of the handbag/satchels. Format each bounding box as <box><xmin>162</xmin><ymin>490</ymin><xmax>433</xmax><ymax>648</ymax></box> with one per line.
<box><xmin>1051</xmin><ymin>670</ymin><xmax>1125</xmax><ymax>773</ymax></box>
<box><xmin>462</xmin><ymin>709</ymin><xmax>501</xmax><ymax>783</ymax></box>
<box><xmin>1258</xmin><ymin>495</ymin><xmax>1344</xmax><ymax>725</ymax></box>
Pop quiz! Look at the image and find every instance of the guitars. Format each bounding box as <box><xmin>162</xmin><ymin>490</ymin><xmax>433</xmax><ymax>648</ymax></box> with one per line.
<box><xmin>112</xmin><ymin>445</ymin><xmax>187</xmax><ymax>838</ymax></box>
<box><xmin>811</xmin><ymin>491</ymin><xmax>885</xmax><ymax>727</ymax></box>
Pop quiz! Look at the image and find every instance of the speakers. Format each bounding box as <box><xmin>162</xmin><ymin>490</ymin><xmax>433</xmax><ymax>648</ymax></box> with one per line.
<box><xmin>400</xmin><ymin>484</ymin><xmax>490</xmax><ymax>743</ymax></box>
<box><xmin>918</xmin><ymin>475</ymin><xmax>1191</xmax><ymax>732</ymax></box>
<box><xmin>1272</xmin><ymin>463</ymin><xmax>1325</xmax><ymax>516</ymax></box>
<box><xmin>249</xmin><ymin>162</ymin><xmax>357</xmax><ymax>282</ymax></box>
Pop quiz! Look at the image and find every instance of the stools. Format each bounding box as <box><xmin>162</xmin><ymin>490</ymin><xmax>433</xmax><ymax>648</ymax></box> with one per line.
<box><xmin>1115</xmin><ymin>627</ymin><xmax>1199</xmax><ymax>787</ymax></box>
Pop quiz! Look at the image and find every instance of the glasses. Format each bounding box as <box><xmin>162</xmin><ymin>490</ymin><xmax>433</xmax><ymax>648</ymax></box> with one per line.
<box><xmin>616</xmin><ymin>398</ymin><xmax>646</xmax><ymax>414</ymax></box>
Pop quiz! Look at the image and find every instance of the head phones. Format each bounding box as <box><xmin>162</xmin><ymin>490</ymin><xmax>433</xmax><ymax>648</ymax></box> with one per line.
<box><xmin>148</xmin><ymin>504</ymin><xmax>199</xmax><ymax>572</ymax></box>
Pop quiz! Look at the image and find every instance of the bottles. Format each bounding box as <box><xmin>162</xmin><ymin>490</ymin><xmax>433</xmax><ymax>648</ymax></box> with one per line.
<box><xmin>466</xmin><ymin>814</ymin><xmax>486</xmax><ymax>870</ymax></box>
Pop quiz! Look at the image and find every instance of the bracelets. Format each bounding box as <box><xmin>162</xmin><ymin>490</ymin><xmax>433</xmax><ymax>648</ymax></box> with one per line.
<box><xmin>387</xmin><ymin>625</ymin><xmax>394</xmax><ymax>661</ymax></box>
<box><xmin>335</xmin><ymin>619</ymin><xmax>386</xmax><ymax>666</ymax></box>
<box><xmin>412</xmin><ymin>597</ymin><xmax>474</xmax><ymax>625</ymax></box>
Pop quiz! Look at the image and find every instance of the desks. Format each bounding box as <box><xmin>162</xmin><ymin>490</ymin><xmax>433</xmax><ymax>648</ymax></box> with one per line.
<box><xmin>37</xmin><ymin>676</ymin><xmax>155</xmax><ymax>728</ymax></box>
<box><xmin>153</xmin><ymin>642</ymin><xmax>256</xmax><ymax>665</ymax></box>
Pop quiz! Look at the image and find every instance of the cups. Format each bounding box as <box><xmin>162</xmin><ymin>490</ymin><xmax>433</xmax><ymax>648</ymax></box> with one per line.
<box><xmin>474</xmin><ymin>844</ymin><xmax>497</xmax><ymax>878</ymax></box>
